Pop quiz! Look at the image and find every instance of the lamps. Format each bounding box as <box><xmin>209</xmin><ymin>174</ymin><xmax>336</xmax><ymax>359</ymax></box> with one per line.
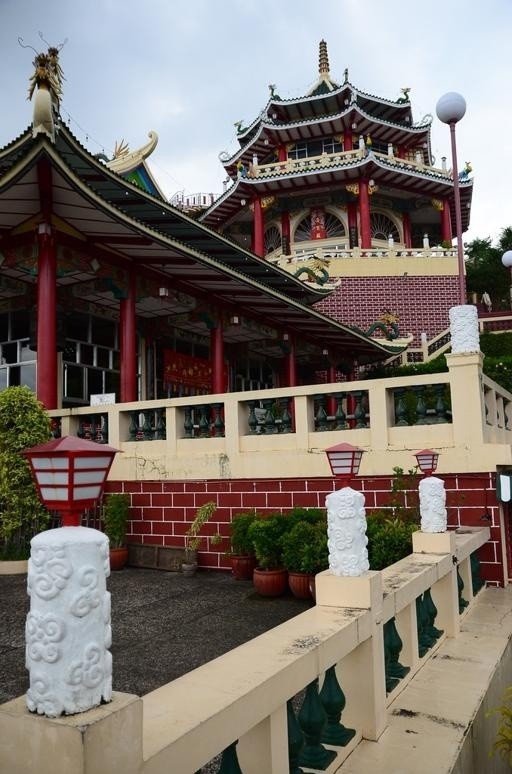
<box><xmin>15</xmin><ymin>433</ymin><xmax>125</xmax><ymax>525</ymax></box>
<box><xmin>322</xmin><ymin>442</ymin><xmax>366</xmax><ymax>488</ymax></box>
<box><xmin>412</xmin><ymin>448</ymin><xmax>440</xmax><ymax>475</ymax></box>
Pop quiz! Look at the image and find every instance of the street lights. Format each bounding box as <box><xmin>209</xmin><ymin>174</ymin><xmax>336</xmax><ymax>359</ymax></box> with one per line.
<box><xmin>434</xmin><ymin>88</ymin><xmax>466</xmax><ymax>306</ymax></box>
<box><xmin>498</xmin><ymin>249</ymin><xmax>512</xmax><ymax>306</ymax></box>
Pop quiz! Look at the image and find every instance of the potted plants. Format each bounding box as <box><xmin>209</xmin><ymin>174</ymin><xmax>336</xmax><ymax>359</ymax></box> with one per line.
<box><xmin>171</xmin><ymin>498</ymin><xmax>219</xmax><ymax>577</ymax></box>
<box><xmin>231</xmin><ymin>509</ymin><xmax>419</xmax><ymax>604</ymax></box>
<box><xmin>100</xmin><ymin>494</ymin><xmax>135</xmax><ymax>571</ymax></box>
